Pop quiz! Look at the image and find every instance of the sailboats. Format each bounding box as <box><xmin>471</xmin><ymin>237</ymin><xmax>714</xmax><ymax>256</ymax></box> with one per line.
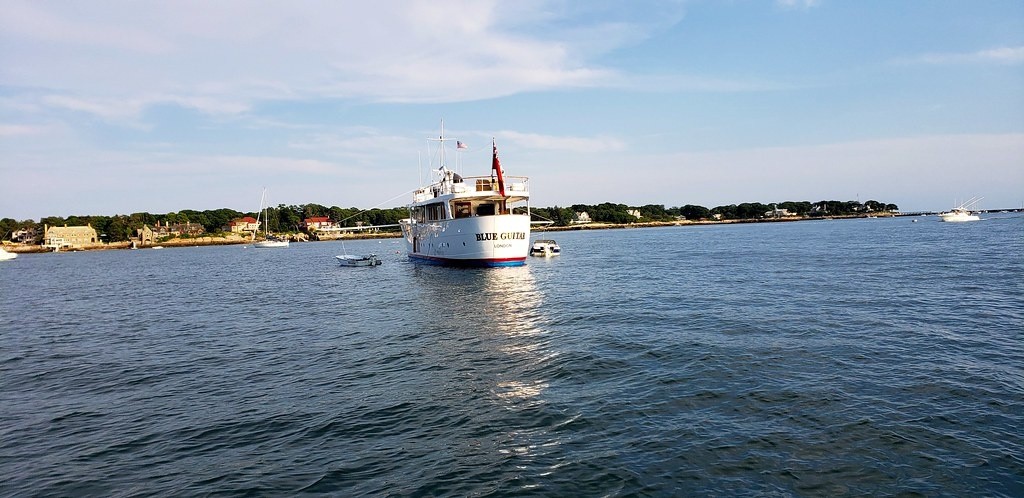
<box><xmin>249</xmin><ymin>186</ymin><xmax>290</xmax><ymax>248</ymax></box>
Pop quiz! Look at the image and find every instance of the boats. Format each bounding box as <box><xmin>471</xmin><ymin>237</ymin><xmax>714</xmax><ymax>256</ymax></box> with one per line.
<box><xmin>530</xmin><ymin>232</ymin><xmax>561</xmax><ymax>258</ymax></box>
<box><xmin>316</xmin><ymin>118</ymin><xmax>554</xmax><ymax>269</ymax></box>
<box><xmin>937</xmin><ymin>196</ymin><xmax>984</xmax><ymax>222</ymax></box>
<box><xmin>336</xmin><ymin>240</ymin><xmax>381</xmax><ymax>268</ymax></box>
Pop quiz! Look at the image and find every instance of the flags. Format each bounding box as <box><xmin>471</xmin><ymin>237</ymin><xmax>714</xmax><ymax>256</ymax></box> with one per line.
<box><xmin>492</xmin><ymin>141</ymin><xmax>504</xmax><ymax>197</ymax></box>
<box><xmin>457</xmin><ymin>141</ymin><xmax>467</xmax><ymax>148</ymax></box>
